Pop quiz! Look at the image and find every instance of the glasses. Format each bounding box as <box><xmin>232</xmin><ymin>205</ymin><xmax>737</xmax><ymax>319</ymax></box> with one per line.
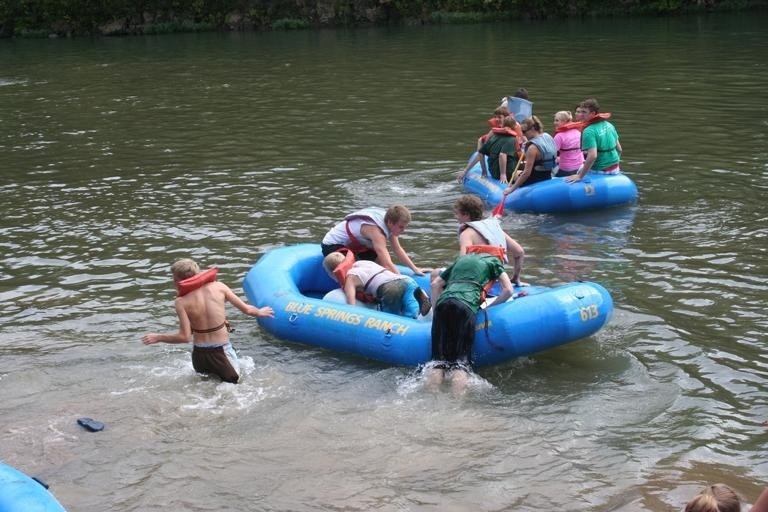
<box><xmin>521</xmin><ymin>126</ymin><xmax>534</xmax><ymax>134</ymax></box>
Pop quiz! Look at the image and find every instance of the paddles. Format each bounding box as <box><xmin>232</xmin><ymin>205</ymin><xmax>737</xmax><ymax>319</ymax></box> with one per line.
<box><xmin>492</xmin><ymin>142</ymin><xmax>524</xmax><ymax>216</ymax></box>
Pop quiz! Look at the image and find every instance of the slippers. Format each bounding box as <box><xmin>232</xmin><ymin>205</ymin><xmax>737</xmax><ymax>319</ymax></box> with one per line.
<box><xmin>77</xmin><ymin>417</ymin><xmax>104</xmax><ymax>431</ymax></box>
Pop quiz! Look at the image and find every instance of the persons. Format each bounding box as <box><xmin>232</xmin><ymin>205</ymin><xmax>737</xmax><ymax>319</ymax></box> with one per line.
<box><xmin>431</xmin><ymin>193</ymin><xmax>531</xmax><ymax>292</ymax></box>
<box><xmin>503</xmin><ymin>115</ymin><xmax>557</xmax><ymax>195</ymax></box>
<box><xmin>574</xmin><ymin>102</ymin><xmax>585</xmax><ymax>122</ymax></box>
<box><xmin>564</xmin><ymin>99</ymin><xmax>623</xmax><ymax>184</ymax></box>
<box><xmin>430</xmin><ymin>247</ymin><xmax>513</xmax><ymax>383</ymax></box>
<box><xmin>477</xmin><ymin>106</ymin><xmax>527</xmax><ymax>177</ymax></box>
<box><xmin>322</xmin><ymin>251</ymin><xmax>431</xmax><ymax>316</ymax></box>
<box><xmin>457</xmin><ymin>116</ymin><xmax>525</xmax><ymax>185</ymax></box>
<box><xmin>501</xmin><ymin>88</ymin><xmax>534</xmax><ymax>125</ymax></box>
<box><xmin>552</xmin><ymin>110</ymin><xmax>586</xmax><ymax>177</ymax></box>
<box><xmin>684</xmin><ymin>483</ymin><xmax>768</xmax><ymax>512</ymax></box>
<box><xmin>321</xmin><ymin>206</ymin><xmax>433</xmax><ymax>276</ymax></box>
<box><xmin>140</xmin><ymin>258</ymin><xmax>275</xmax><ymax>383</ymax></box>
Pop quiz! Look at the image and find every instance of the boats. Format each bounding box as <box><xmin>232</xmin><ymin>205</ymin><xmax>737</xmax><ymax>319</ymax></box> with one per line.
<box><xmin>242</xmin><ymin>243</ymin><xmax>613</xmax><ymax>371</ymax></box>
<box><xmin>466</xmin><ymin>150</ymin><xmax>637</xmax><ymax>214</ymax></box>
